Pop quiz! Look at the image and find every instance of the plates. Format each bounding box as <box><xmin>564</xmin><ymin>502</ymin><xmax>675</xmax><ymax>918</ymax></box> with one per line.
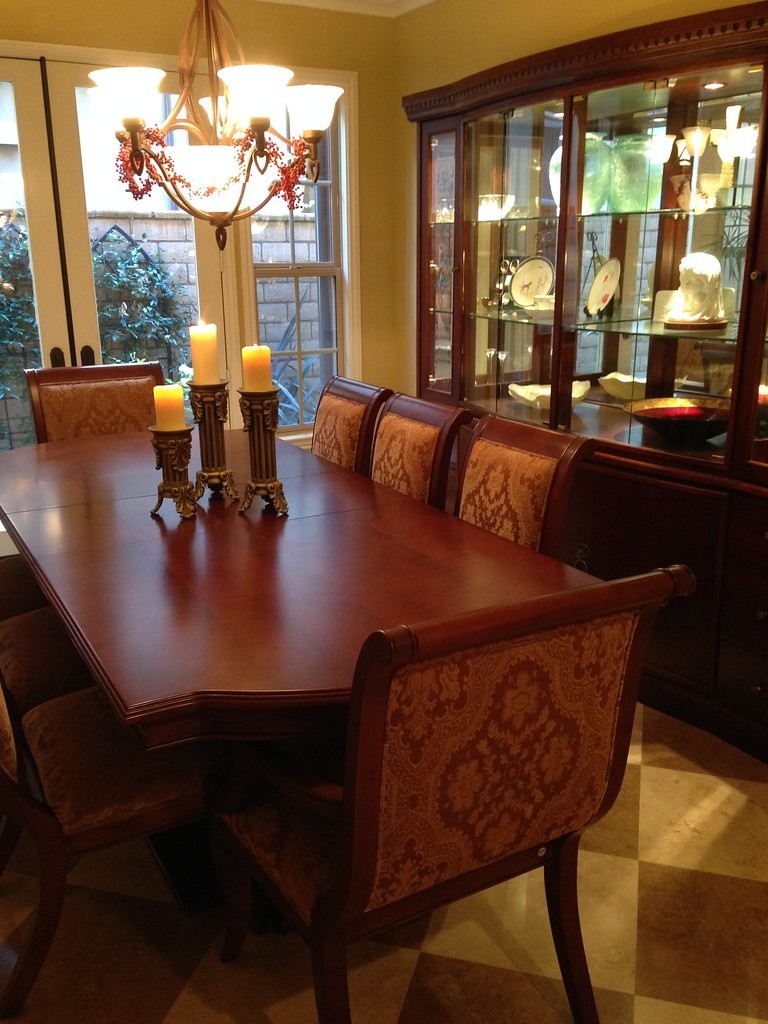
<box><xmin>587</xmin><ymin>257</ymin><xmax>621</xmax><ymax>314</ymax></box>
<box><xmin>510</xmin><ymin>257</ymin><xmax>554</xmax><ymax>306</ymax></box>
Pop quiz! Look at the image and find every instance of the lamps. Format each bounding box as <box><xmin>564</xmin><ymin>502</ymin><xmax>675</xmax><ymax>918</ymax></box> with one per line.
<box><xmin>87</xmin><ymin>1</ymin><xmax>345</xmax><ymax>250</ymax></box>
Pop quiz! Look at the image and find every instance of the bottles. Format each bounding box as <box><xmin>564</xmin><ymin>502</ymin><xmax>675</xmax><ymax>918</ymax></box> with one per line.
<box><xmin>548</xmin><ymin>132</ymin><xmax>615</xmax><ymax>216</ymax></box>
<box><xmin>608</xmin><ymin>134</ymin><xmax>663</xmax><ymax>212</ymax></box>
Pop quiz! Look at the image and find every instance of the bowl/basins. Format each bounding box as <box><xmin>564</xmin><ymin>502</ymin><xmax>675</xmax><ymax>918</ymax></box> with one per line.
<box><xmin>478</xmin><ymin>194</ymin><xmax>515</xmax><ymax>220</ymax></box>
<box><xmin>598</xmin><ymin>372</ymin><xmax>688</xmax><ymax>400</ymax></box>
<box><xmin>622</xmin><ymin>397</ymin><xmax>731</xmax><ymax>441</ymax></box>
<box><xmin>508</xmin><ymin>380</ymin><xmax>590</xmax><ymax>410</ymax></box>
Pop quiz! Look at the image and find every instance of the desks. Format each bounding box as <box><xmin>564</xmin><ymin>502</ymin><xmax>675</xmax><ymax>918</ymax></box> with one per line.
<box><xmin>1</xmin><ymin>428</ymin><xmax>608</xmax><ymax>967</ymax></box>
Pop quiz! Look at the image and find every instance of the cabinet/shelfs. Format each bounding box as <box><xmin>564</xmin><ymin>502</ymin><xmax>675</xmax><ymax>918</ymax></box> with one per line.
<box><xmin>395</xmin><ymin>0</ymin><xmax>767</xmax><ymax>763</ymax></box>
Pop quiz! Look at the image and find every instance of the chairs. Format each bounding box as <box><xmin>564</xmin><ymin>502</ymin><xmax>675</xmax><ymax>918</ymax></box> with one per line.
<box><xmin>0</xmin><ymin>360</ymin><xmax>692</xmax><ymax>1024</ymax></box>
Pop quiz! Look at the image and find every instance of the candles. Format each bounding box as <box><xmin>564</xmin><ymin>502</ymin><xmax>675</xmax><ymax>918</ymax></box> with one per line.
<box><xmin>242</xmin><ymin>343</ymin><xmax>273</xmax><ymax>392</ymax></box>
<box><xmin>189</xmin><ymin>318</ymin><xmax>221</xmax><ymax>385</ymax></box>
<box><xmin>153</xmin><ymin>384</ymin><xmax>185</xmax><ymax>431</ymax></box>
